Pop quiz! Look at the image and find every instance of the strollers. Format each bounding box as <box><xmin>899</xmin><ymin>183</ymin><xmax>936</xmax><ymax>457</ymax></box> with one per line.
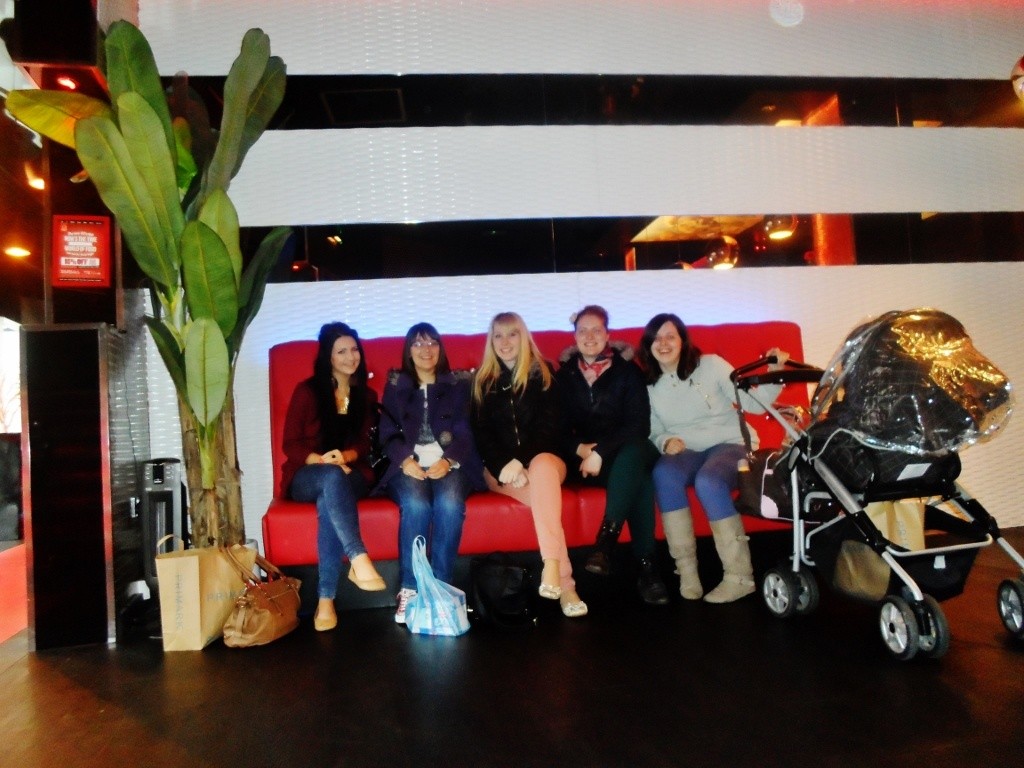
<box><xmin>732</xmin><ymin>307</ymin><xmax>1024</xmax><ymax>659</ymax></box>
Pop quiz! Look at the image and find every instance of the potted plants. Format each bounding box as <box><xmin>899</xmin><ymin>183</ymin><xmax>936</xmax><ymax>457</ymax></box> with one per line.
<box><xmin>3</xmin><ymin>19</ymin><xmax>296</xmax><ymax>651</ymax></box>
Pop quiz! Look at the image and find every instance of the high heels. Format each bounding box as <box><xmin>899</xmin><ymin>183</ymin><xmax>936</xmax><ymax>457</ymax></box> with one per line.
<box><xmin>313</xmin><ymin>598</ymin><xmax>337</xmax><ymax>632</ymax></box>
<box><xmin>348</xmin><ymin>564</ymin><xmax>386</xmax><ymax>591</ymax></box>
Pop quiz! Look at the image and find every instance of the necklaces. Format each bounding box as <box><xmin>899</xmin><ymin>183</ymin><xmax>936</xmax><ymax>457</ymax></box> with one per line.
<box><xmin>337</xmin><ymin>393</ymin><xmax>349</xmax><ymax>412</ymax></box>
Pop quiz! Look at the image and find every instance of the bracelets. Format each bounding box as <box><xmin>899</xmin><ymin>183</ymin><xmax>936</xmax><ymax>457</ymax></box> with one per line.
<box><xmin>444</xmin><ymin>457</ymin><xmax>452</xmax><ymax>472</ymax></box>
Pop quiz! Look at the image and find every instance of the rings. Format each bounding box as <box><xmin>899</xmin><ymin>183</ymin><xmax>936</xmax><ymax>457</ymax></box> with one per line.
<box><xmin>332</xmin><ymin>455</ymin><xmax>336</xmax><ymax>458</ymax></box>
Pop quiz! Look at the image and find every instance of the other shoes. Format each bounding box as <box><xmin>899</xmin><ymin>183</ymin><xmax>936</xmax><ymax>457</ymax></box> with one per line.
<box><xmin>538</xmin><ymin>568</ymin><xmax>560</xmax><ymax>599</ymax></box>
<box><xmin>559</xmin><ymin>594</ymin><xmax>588</xmax><ymax>616</ymax></box>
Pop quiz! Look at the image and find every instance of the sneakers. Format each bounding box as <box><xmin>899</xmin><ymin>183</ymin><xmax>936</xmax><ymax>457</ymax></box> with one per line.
<box><xmin>394</xmin><ymin>588</ymin><xmax>418</xmax><ymax>624</ymax></box>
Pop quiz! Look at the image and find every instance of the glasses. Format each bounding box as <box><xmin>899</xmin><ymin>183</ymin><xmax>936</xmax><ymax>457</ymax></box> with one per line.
<box><xmin>413</xmin><ymin>339</ymin><xmax>440</xmax><ymax>349</ymax></box>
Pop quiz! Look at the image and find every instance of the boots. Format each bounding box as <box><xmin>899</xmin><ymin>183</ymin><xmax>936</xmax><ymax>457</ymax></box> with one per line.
<box><xmin>635</xmin><ymin>539</ymin><xmax>667</xmax><ymax>604</ymax></box>
<box><xmin>661</xmin><ymin>506</ymin><xmax>705</xmax><ymax>600</ymax></box>
<box><xmin>704</xmin><ymin>514</ymin><xmax>757</xmax><ymax>603</ymax></box>
<box><xmin>586</xmin><ymin>516</ymin><xmax>625</xmax><ymax>574</ymax></box>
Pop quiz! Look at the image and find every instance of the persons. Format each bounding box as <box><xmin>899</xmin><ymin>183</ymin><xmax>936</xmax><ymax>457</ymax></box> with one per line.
<box><xmin>471</xmin><ymin>312</ymin><xmax>588</xmax><ymax>618</ymax></box>
<box><xmin>637</xmin><ymin>313</ymin><xmax>789</xmax><ymax>604</ymax></box>
<box><xmin>280</xmin><ymin>321</ymin><xmax>387</xmax><ymax>632</ymax></box>
<box><xmin>555</xmin><ymin>304</ymin><xmax>667</xmax><ymax>604</ymax></box>
<box><xmin>377</xmin><ymin>323</ymin><xmax>485</xmax><ymax>624</ymax></box>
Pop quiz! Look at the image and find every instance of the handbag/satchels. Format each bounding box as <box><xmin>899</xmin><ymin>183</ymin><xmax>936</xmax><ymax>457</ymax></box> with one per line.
<box><xmin>369</xmin><ymin>401</ymin><xmax>405</xmax><ymax>472</ymax></box>
<box><xmin>218</xmin><ymin>538</ymin><xmax>305</xmax><ymax>648</ymax></box>
<box><xmin>736</xmin><ymin>447</ymin><xmax>783</xmax><ymax>516</ymax></box>
<box><xmin>405</xmin><ymin>534</ymin><xmax>472</xmax><ymax>636</ymax></box>
<box><xmin>155</xmin><ymin>533</ymin><xmax>259</xmax><ymax>651</ymax></box>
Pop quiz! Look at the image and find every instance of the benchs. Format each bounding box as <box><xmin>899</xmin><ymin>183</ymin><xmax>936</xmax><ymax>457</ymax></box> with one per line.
<box><xmin>262</xmin><ymin>315</ymin><xmax>817</xmax><ymax>590</ymax></box>
